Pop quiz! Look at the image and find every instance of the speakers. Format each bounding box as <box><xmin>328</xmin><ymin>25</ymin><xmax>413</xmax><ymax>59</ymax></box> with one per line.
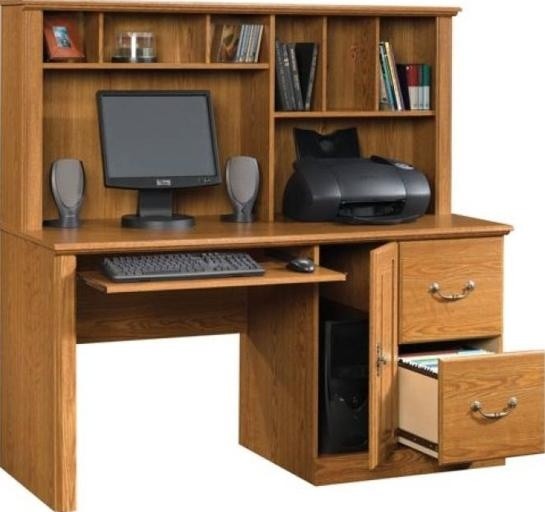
<box><xmin>225</xmin><ymin>156</ymin><xmax>259</xmax><ymax>223</ymax></box>
<box><xmin>50</xmin><ymin>158</ymin><xmax>87</xmax><ymax>229</ymax></box>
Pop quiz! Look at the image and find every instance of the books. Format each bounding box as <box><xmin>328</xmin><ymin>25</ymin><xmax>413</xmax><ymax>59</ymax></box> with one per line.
<box><xmin>379</xmin><ymin>41</ymin><xmax>434</xmax><ymax>110</ymax></box>
<box><xmin>215</xmin><ymin>19</ymin><xmax>320</xmax><ymax>112</ymax></box>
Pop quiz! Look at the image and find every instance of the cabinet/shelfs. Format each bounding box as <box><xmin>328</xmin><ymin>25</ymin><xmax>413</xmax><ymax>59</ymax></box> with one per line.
<box><xmin>0</xmin><ymin>0</ymin><xmax>464</xmax><ymax>235</ymax></box>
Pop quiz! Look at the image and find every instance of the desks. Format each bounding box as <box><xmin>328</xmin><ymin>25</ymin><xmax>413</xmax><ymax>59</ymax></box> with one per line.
<box><xmin>0</xmin><ymin>214</ymin><xmax>545</xmax><ymax>510</ymax></box>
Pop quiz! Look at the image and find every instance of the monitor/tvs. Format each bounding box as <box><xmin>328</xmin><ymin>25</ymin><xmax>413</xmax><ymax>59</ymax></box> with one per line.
<box><xmin>95</xmin><ymin>89</ymin><xmax>221</xmax><ymax>230</ymax></box>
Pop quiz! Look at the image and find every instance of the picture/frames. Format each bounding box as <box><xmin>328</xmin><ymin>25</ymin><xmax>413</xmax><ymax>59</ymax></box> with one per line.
<box><xmin>41</xmin><ymin>15</ymin><xmax>87</xmax><ymax>63</ymax></box>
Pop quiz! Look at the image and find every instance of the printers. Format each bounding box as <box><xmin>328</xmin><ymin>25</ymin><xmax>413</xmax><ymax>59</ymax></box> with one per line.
<box><xmin>282</xmin><ymin>127</ymin><xmax>431</xmax><ymax>225</ymax></box>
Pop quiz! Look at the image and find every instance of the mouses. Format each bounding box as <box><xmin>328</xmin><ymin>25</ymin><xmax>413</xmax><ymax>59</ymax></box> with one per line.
<box><xmin>290</xmin><ymin>257</ymin><xmax>314</xmax><ymax>271</ymax></box>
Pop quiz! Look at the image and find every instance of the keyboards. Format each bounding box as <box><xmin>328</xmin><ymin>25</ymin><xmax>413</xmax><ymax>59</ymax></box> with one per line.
<box><xmin>104</xmin><ymin>250</ymin><xmax>265</xmax><ymax>282</ymax></box>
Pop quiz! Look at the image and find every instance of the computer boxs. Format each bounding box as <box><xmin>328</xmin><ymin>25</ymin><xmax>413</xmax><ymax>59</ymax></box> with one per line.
<box><xmin>319</xmin><ymin>295</ymin><xmax>368</xmax><ymax>453</ymax></box>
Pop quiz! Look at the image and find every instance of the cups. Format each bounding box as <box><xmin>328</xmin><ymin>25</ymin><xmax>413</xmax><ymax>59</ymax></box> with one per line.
<box><xmin>114</xmin><ymin>30</ymin><xmax>158</xmax><ymax>62</ymax></box>
